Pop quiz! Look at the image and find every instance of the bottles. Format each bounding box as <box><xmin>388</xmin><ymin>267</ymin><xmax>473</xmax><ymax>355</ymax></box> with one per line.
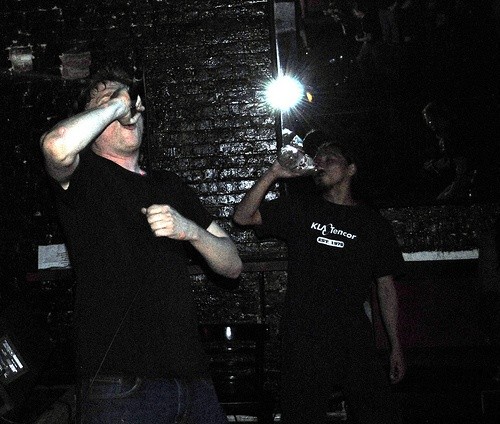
<box><xmin>276</xmin><ymin>144</ymin><xmax>323</xmax><ymax>176</ymax></box>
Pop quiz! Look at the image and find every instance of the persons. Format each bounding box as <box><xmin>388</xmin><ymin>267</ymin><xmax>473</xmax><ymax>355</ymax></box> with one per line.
<box><xmin>232</xmin><ymin>134</ymin><xmax>408</xmax><ymax>424</ymax></box>
<box><xmin>28</xmin><ymin>65</ymin><xmax>248</xmax><ymax>424</ymax></box>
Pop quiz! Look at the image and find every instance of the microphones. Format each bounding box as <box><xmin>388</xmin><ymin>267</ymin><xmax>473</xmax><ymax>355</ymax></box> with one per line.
<box><xmin>128</xmin><ymin>66</ymin><xmax>144</xmax><ymax>126</ymax></box>
